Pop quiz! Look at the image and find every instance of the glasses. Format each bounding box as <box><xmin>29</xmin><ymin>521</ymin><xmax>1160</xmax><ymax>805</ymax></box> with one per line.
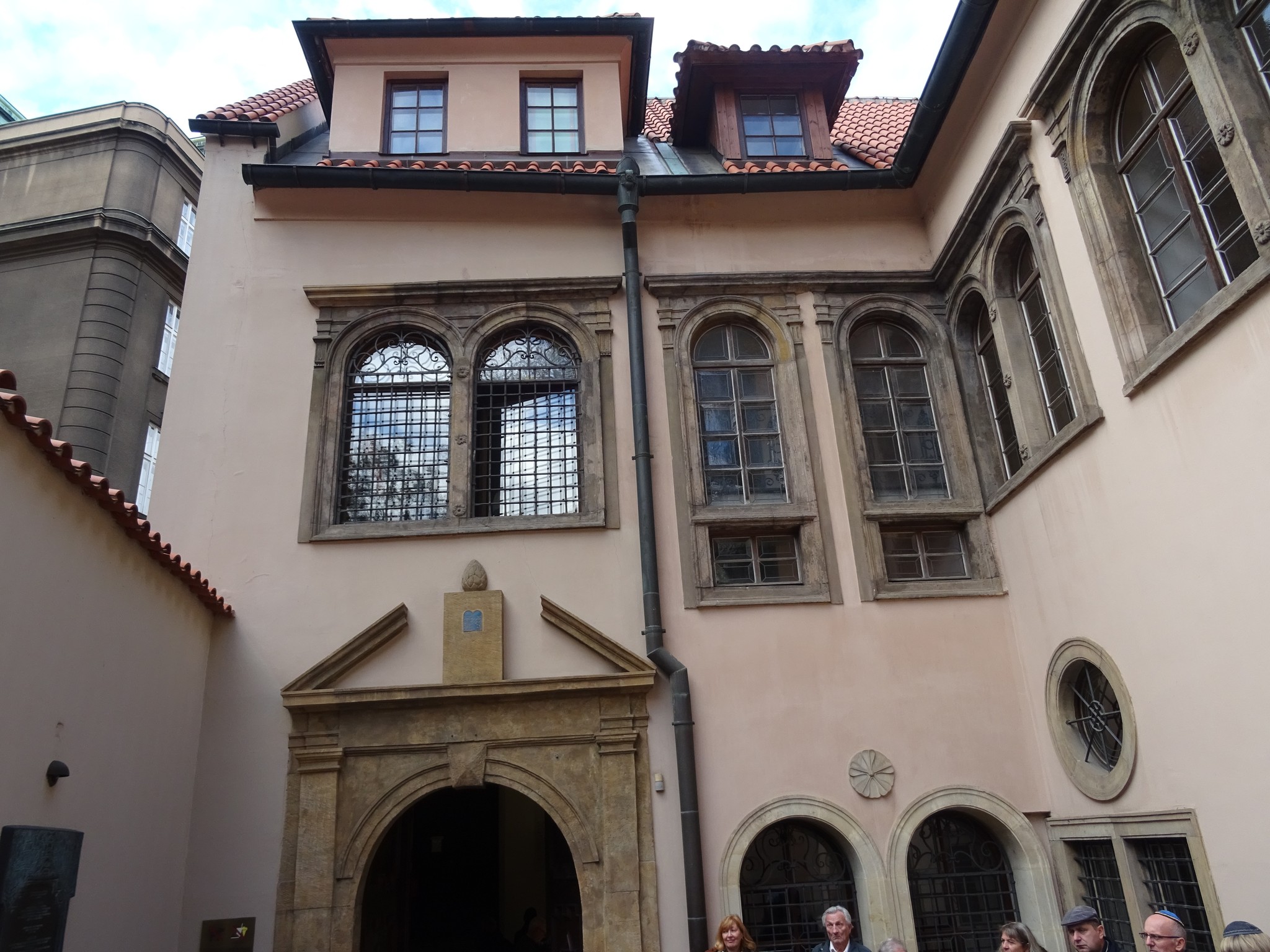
<box><xmin>1139</xmin><ymin>933</ymin><xmax>1180</xmax><ymax>940</ymax></box>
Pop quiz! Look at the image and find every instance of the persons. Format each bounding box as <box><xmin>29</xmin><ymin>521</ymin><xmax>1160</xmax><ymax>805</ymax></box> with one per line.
<box><xmin>878</xmin><ymin>938</ymin><xmax>908</xmax><ymax>952</ymax></box>
<box><xmin>811</xmin><ymin>906</ymin><xmax>873</xmax><ymax>952</ymax></box>
<box><xmin>997</xmin><ymin>921</ymin><xmax>1048</xmax><ymax>952</ymax></box>
<box><xmin>705</xmin><ymin>913</ymin><xmax>757</xmax><ymax>952</ymax></box>
<box><xmin>512</xmin><ymin>916</ymin><xmax>547</xmax><ymax>952</ymax></box>
<box><xmin>1061</xmin><ymin>905</ymin><xmax>1131</xmax><ymax>952</ymax></box>
<box><xmin>1139</xmin><ymin>910</ymin><xmax>1187</xmax><ymax>952</ymax></box>
<box><xmin>1220</xmin><ymin>921</ymin><xmax>1270</xmax><ymax>952</ymax></box>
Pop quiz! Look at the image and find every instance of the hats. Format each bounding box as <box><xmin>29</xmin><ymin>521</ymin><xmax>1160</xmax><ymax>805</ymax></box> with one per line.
<box><xmin>1223</xmin><ymin>921</ymin><xmax>1264</xmax><ymax>938</ymax></box>
<box><xmin>1061</xmin><ymin>905</ymin><xmax>1100</xmax><ymax>926</ymax></box>
<box><xmin>1153</xmin><ymin>910</ymin><xmax>1186</xmax><ymax>929</ymax></box>
